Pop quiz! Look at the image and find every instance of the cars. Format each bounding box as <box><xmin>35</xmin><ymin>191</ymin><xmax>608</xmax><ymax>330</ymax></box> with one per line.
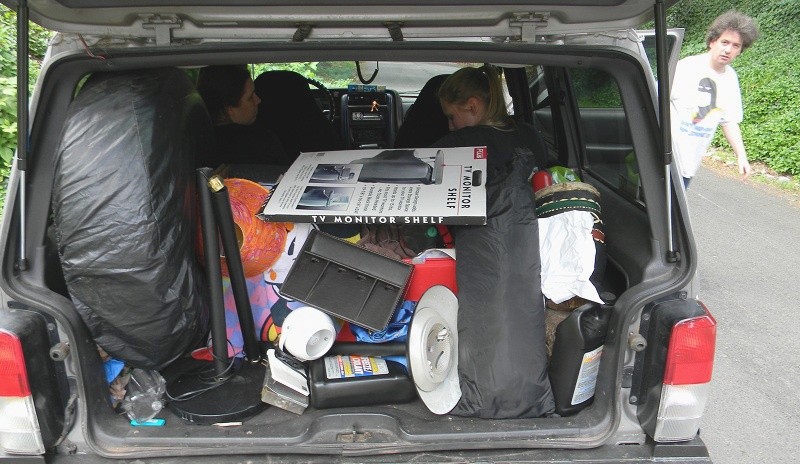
<box><xmin>0</xmin><ymin>0</ymin><xmax>720</xmax><ymax>464</ymax></box>
<box><xmin>362</xmin><ymin>35</ymin><xmax>512</xmax><ymax>102</ymax></box>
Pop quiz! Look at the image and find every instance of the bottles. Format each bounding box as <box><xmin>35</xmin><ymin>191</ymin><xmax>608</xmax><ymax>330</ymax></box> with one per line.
<box><xmin>403</xmin><ymin>225</ymin><xmax>437</xmax><ymax>255</ymax></box>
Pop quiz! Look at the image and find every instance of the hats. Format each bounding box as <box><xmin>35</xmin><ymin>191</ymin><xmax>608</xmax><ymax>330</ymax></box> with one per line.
<box><xmin>194</xmin><ymin>177</ymin><xmax>287</xmax><ymax>277</ymax></box>
<box><xmin>408</xmin><ymin>285</ymin><xmax>462</xmax><ymax>417</ymax></box>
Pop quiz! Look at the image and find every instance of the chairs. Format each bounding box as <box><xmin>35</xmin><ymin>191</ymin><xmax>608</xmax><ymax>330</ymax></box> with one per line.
<box><xmin>394</xmin><ymin>73</ymin><xmax>451</xmax><ymax>147</ymax></box>
<box><xmin>253</xmin><ymin>70</ymin><xmax>341</xmax><ymax>166</ymax></box>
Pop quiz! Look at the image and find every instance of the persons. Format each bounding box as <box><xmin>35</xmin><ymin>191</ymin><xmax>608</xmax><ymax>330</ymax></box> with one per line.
<box><xmin>427</xmin><ymin>65</ymin><xmax>549</xmax><ymax>186</ymax></box>
<box><xmin>658</xmin><ymin>9</ymin><xmax>757</xmax><ymax>191</ymax></box>
<box><xmin>195</xmin><ymin>63</ymin><xmax>276</xmax><ymax>184</ymax></box>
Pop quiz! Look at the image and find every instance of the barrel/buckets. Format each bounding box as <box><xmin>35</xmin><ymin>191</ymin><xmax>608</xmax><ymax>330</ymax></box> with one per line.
<box><xmin>550</xmin><ymin>292</ymin><xmax>617</xmax><ymax>417</ymax></box>
<box><xmin>306</xmin><ymin>355</ymin><xmax>415</xmax><ymax>409</ymax></box>
